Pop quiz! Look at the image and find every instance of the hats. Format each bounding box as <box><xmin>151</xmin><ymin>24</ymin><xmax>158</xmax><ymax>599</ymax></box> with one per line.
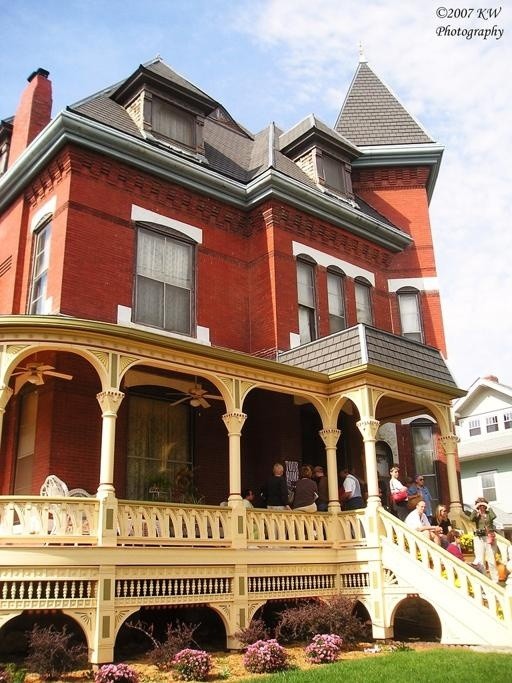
<box><xmin>474</xmin><ymin>497</ymin><xmax>488</xmax><ymax>510</ymax></box>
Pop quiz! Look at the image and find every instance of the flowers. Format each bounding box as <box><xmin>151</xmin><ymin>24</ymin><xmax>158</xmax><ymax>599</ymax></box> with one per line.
<box><xmin>457</xmin><ymin>532</ymin><xmax>475</xmax><ymax>555</ymax></box>
<box><xmin>483</xmin><ymin>548</ymin><xmax>502</xmax><ymax>566</ymax></box>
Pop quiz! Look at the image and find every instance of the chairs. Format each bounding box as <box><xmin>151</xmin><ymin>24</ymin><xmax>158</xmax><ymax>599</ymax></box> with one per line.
<box><xmin>64</xmin><ymin>487</ymin><xmax>97</xmax><ymax>535</ymax></box>
<box><xmin>40</xmin><ymin>473</ymin><xmax>95</xmax><ymax>548</ymax></box>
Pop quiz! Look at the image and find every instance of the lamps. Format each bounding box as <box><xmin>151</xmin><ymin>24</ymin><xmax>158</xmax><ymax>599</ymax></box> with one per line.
<box><xmin>188</xmin><ymin>395</ymin><xmax>203</xmax><ymax>408</ymax></box>
<box><xmin>27</xmin><ymin>370</ymin><xmax>42</xmax><ymax>384</ymax></box>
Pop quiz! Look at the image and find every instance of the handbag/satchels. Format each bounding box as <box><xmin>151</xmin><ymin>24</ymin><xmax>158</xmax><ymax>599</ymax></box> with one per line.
<box><xmin>405</xmin><ymin>484</ymin><xmax>424</xmax><ymax>514</ymax></box>
<box><xmin>487</xmin><ymin>531</ymin><xmax>496</xmax><ymax>544</ymax></box>
<box><xmin>390</xmin><ymin>477</ymin><xmax>408</xmax><ymax>503</ymax></box>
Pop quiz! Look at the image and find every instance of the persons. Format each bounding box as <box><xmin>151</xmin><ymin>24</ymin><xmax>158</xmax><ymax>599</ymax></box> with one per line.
<box><xmin>292</xmin><ymin>463</ymin><xmax>319</xmax><ymax>513</ymax></box>
<box><xmin>377</xmin><ymin>469</ymin><xmax>388</xmax><ymax>510</ymax></box>
<box><xmin>431</xmin><ymin>503</ymin><xmax>453</xmax><ymax>538</ymax></box>
<box><xmin>446</xmin><ymin>529</ymin><xmax>466</xmax><ymax>560</ymax></box>
<box><xmin>241</xmin><ymin>487</ymin><xmax>256</xmax><ymax>501</ymax></box>
<box><xmin>407</xmin><ymin>473</ymin><xmax>433</xmax><ymax>525</ymax></box>
<box><xmin>339</xmin><ymin>469</ymin><xmax>363</xmax><ymax>511</ymax></box>
<box><xmin>313</xmin><ymin>465</ymin><xmax>329</xmax><ymax>510</ymax></box>
<box><xmin>262</xmin><ymin>462</ymin><xmax>292</xmax><ymax>510</ymax></box>
<box><xmin>388</xmin><ymin>466</ymin><xmax>411</xmax><ymax>521</ymax></box>
<box><xmin>470</xmin><ymin>497</ymin><xmax>500</xmax><ymax>583</ymax></box>
<box><xmin>403</xmin><ymin>500</ymin><xmax>444</xmax><ymax>546</ymax></box>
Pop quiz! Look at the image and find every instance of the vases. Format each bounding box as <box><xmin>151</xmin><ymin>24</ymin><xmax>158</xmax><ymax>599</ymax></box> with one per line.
<box><xmin>494</xmin><ymin>560</ymin><xmax>506</xmax><ymax>582</ymax></box>
<box><xmin>460</xmin><ymin>552</ymin><xmax>476</xmax><ymax>563</ymax></box>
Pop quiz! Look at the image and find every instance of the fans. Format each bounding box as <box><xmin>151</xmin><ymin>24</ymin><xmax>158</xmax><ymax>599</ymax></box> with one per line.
<box><xmin>10</xmin><ymin>349</ymin><xmax>76</xmax><ymax>396</ymax></box>
<box><xmin>166</xmin><ymin>373</ymin><xmax>225</xmax><ymax>408</ymax></box>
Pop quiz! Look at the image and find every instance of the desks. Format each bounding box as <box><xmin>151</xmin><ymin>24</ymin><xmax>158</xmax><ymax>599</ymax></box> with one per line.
<box><xmin>115</xmin><ymin>514</ymin><xmax>164</xmax><ymax>549</ymax></box>
